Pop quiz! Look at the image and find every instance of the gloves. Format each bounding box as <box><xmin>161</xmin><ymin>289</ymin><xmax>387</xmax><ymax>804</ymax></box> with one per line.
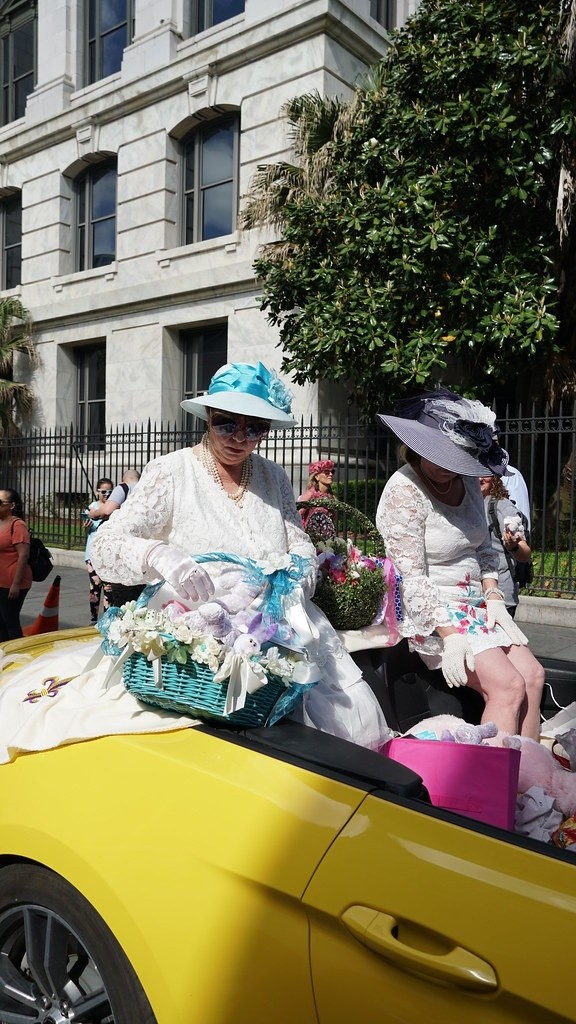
<box><xmin>441</xmin><ymin>633</ymin><xmax>475</xmax><ymax>688</ymax></box>
<box><xmin>146</xmin><ymin>544</ymin><xmax>215</xmax><ymax>602</ymax></box>
<box><xmin>485</xmin><ymin>599</ymin><xmax>529</xmax><ymax>646</ymax></box>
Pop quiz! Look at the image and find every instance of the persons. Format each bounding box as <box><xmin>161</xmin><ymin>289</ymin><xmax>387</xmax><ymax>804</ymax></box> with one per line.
<box><xmin>501</xmin><ymin>466</ymin><xmax>530</xmax><ymax>530</ymax></box>
<box><xmin>82</xmin><ymin>470</ymin><xmax>141</xmax><ymax>625</ymax></box>
<box><xmin>0</xmin><ymin>488</ymin><xmax>32</xmax><ymax>642</ymax></box>
<box><xmin>375</xmin><ymin>400</ymin><xmax>545</xmax><ymax>744</ymax></box>
<box><xmin>478</xmin><ymin>477</ymin><xmax>531</xmax><ymax>619</ymax></box>
<box><xmin>90</xmin><ymin>364</ymin><xmax>389</xmax><ymax>752</ymax></box>
<box><xmin>299</xmin><ymin>461</ymin><xmax>338</xmax><ymax>527</ymax></box>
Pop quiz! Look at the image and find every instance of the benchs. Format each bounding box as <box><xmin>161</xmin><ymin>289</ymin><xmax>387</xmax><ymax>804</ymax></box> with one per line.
<box><xmin>353</xmin><ymin>641</ymin><xmax>486</xmax><ymax>733</ymax></box>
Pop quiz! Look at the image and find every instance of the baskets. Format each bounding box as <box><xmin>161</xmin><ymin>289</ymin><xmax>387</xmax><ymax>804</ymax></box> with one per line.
<box><xmin>295</xmin><ymin>499</ymin><xmax>387</xmax><ymax>630</ymax></box>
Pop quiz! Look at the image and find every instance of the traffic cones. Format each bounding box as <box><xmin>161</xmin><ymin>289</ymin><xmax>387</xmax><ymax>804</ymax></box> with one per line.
<box><xmin>19</xmin><ymin>574</ymin><xmax>62</xmax><ymax>637</ymax></box>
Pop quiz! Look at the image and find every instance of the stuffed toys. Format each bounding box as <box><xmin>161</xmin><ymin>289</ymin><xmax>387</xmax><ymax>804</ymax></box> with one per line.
<box><xmin>403</xmin><ymin>715</ymin><xmax>576</xmax><ymax>817</ymax></box>
<box><xmin>164</xmin><ymin>569</ymin><xmax>279</xmax><ymax>659</ymax></box>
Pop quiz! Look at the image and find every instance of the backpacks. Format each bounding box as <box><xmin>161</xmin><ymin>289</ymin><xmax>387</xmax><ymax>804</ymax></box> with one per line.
<box><xmin>487</xmin><ymin>496</ymin><xmax>534</xmax><ymax>589</ymax></box>
<box><xmin>11</xmin><ymin>518</ymin><xmax>54</xmax><ymax>582</ymax></box>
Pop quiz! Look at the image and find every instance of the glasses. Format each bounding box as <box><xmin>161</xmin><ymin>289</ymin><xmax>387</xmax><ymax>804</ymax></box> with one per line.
<box><xmin>320</xmin><ymin>467</ymin><xmax>335</xmax><ymax>476</ymax></box>
<box><xmin>97</xmin><ymin>488</ymin><xmax>112</xmax><ymax>495</ymax></box>
<box><xmin>210</xmin><ymin>408</ymin><xmax>272</xmax><ymax>442</ymax></box>
<box><xmin>0</xmin><ymin>500</ymin><xmax>15</xmax><ymax>507</ymax></box>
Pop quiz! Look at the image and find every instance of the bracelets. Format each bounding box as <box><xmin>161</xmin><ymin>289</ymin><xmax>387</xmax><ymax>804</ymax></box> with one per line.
<box><xmin>485</xmin><ymin>588</ymin><xmax>505</xmax><ymax>600</ymax></box>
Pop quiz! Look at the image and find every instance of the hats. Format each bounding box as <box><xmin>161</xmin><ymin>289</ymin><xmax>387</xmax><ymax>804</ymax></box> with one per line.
<box><xmin>309</xmin><ymin>460</ymin><xmax>335</xmax><ymax>475</ymax></box>
<box><xmin>376</xmin><ymin>391</ymin><xmax>510</xmax><ymax>478</ymax></box>
<box><xmin>179</xmin><ymin>362</ymin><xmax>298</xmax><ymax>430</ymax></box>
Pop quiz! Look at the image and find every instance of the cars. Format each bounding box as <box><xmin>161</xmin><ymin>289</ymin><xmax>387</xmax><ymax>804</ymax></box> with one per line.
<box><xmin>0</xmin><ymin>629</ymin><xmax>576</xmax><ymax>1023</ymax></box>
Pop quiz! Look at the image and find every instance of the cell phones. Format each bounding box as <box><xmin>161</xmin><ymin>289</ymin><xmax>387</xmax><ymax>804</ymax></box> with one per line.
<box><xmin>81</xmin><ymin>513</ymin><xmax>91</xmax><ymax>522</ymax></box>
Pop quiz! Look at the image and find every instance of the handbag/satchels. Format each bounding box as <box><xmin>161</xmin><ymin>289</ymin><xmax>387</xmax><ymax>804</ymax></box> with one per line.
<box><xmin>377</xmin><ymin>739</ymin><xmax>522</xmax><ymax>829</ymax></box>
<box><xmin>120</xmin><ymin>555</ymin><xmax>290</xmax><ymax>729</ymax></box>
<box><xmin>537</xmin><ymin>682</ymin><xmax>576</xmax><ymax>743</ymax></box>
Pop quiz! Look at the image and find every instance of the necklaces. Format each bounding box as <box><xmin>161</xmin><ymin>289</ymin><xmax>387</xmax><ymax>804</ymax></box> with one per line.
<box><xmin>420</xmin><ymin>464</ymin><xmax>453</xmax><ymax>494</ymax></box>
<box><xmin>200</xmin><ymin>433</ymin><xmax>252</xmax><ymax>508</ymax></box>
